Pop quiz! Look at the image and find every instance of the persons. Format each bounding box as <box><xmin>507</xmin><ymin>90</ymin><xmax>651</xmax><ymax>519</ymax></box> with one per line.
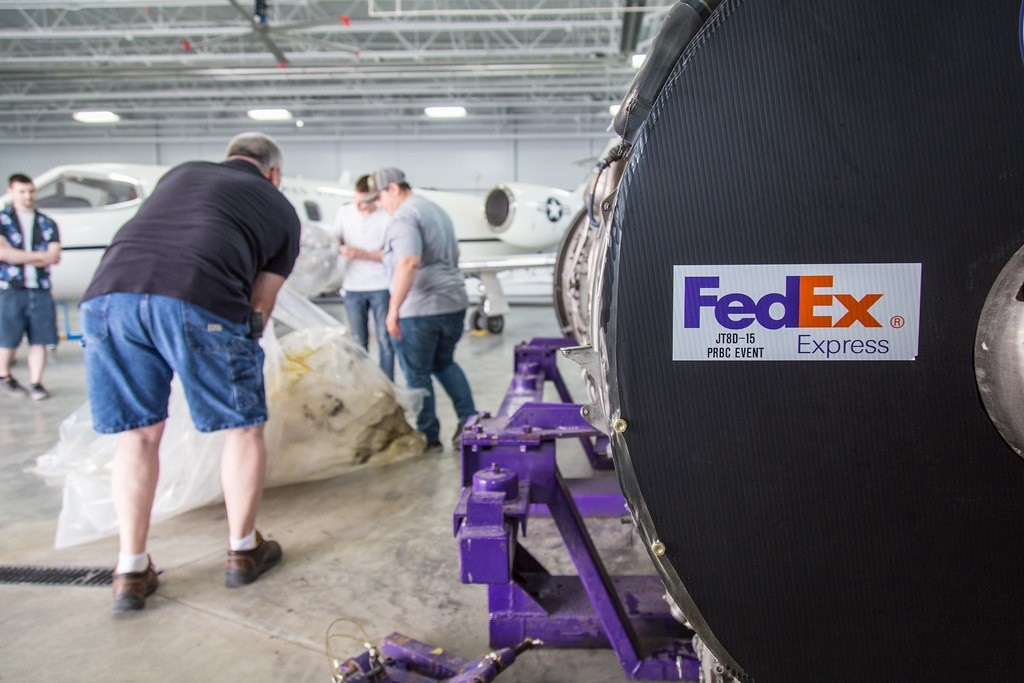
<box><xmin>0</xmin><ymin>175</ymin><xmax>61</xmax><ymax>399</ymax></box>
<box><xmin>336</xmin><ymin>175</ymin><xmax>396</xmax><ymax>384</ymax></box>
<box><xmin>77</xmin><ymin>132</ymin><xmax>301</xmax><ymax>610</ymax></box>
<box><xmin>362</xmin><ymin>166</ymin><xmax>477</xmax><ymax>452</ymax></box>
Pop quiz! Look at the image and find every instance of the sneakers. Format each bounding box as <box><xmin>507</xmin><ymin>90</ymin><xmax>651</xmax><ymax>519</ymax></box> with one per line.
<box><xmin>225</xmin><ymin>530</ymin><xmax>282</xmax><ymax>588</ymax></box>
<box><xmin>113</xmin><ymin>554</ymin><xmax>163</xmax><ymax>609</ymax></box>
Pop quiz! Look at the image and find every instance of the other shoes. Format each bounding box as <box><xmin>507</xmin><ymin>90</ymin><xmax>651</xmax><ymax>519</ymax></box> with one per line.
<box><xmin>0</xmin><ymin>375</ymin><xmax>27</xmax><ymax>397</ymax></box>
<box><xmin>453</xmin><ymin>421</ymin><xmax>462</xmax><ymax>451</ymax></box>
<box><xmin>425</xmin><ymin>438</ymin><xmax>442</xmax><ymax>453</ymax></box>
<box><xmin>28</xmin><ymin>384</ymin><xmax>48</xmax><ymax>400</ymax></box>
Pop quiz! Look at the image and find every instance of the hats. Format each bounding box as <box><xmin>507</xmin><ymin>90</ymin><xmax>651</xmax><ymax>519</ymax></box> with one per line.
<box><xmin>363</xmin><ymin>167</ymin><xmax>407</xmax><ymax>201</ymax></box>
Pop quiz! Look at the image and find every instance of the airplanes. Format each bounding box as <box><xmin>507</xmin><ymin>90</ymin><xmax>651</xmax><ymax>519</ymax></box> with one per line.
<box><xmin>1</xmin><ymin>165</ymin><xmax>586</xmax><ymax>335</ymax></box>
